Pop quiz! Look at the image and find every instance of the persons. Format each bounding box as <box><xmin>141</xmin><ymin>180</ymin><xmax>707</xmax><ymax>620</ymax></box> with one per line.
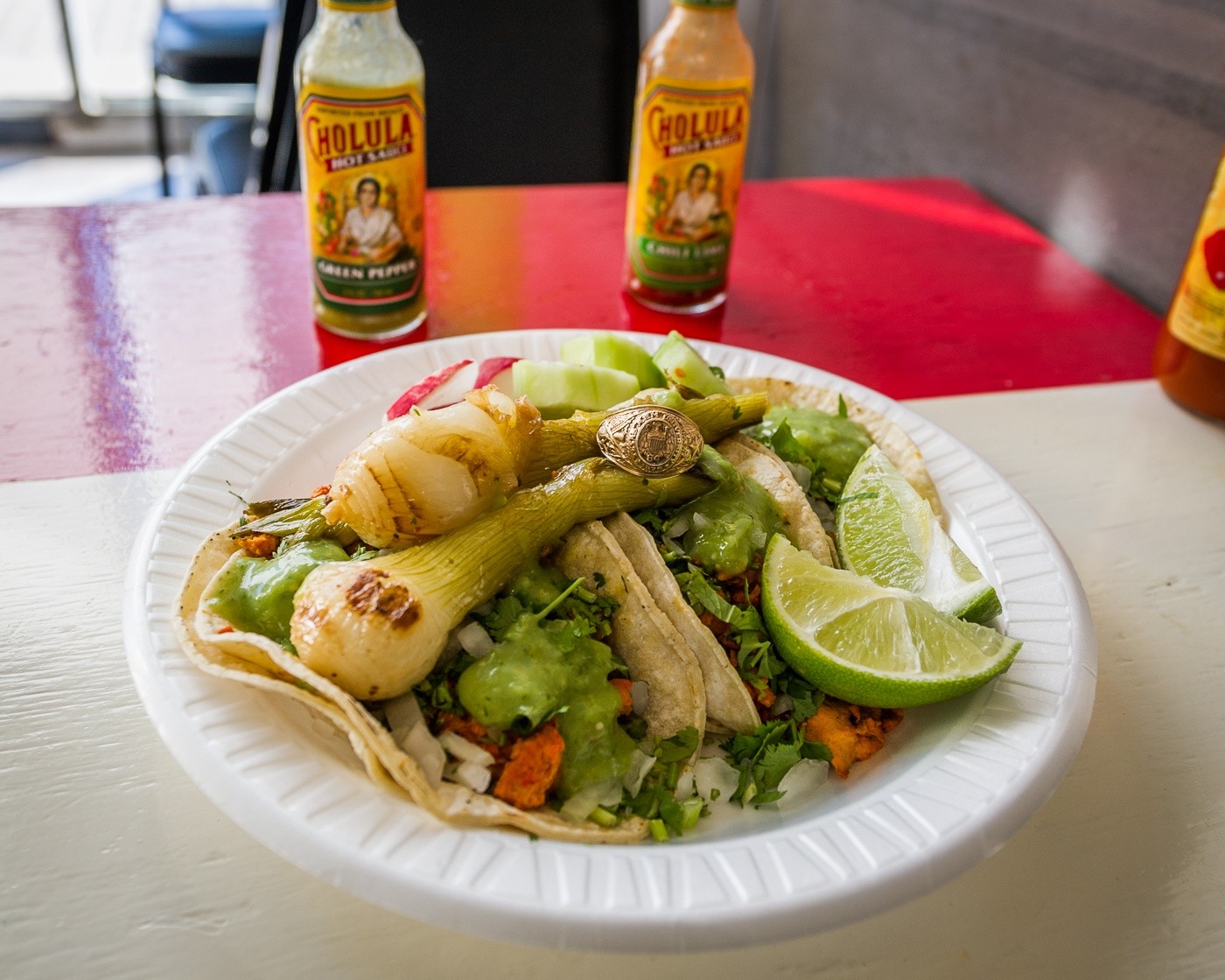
<box><xmin>662</xmin><ymin>163</ymin><xmax>720</xmax><ymax>242</ymax></box>
<box><xmin>338</xmin><ymin>176</ymin><xmax>405</xmax><ymax>259</ymax></box>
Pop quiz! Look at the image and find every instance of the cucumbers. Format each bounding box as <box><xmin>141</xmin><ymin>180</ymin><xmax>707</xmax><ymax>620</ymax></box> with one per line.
<box><xmin>506</xmin><ymin>332</ymin><xmax>734</xmax><ymax>408</ymax></box>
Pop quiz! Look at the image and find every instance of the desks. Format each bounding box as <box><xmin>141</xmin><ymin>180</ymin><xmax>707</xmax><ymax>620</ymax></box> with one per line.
<box><xmin>0</xmin><ymin>175</ymin><xmax>1225</xmax><ymax>980</ymax></box>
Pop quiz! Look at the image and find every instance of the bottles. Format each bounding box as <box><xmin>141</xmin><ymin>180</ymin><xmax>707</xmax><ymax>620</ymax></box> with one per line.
<box><xmin>624</xmin><ymin>0</ymin><xmax>755</xmax><ymax>315</ymax></box>
<box><xmin>291</xmin><ymin>0</ymin><xmax>429</xmax><ymax>342</ymax></box>
<box><xmin>1153</xmin><ymin>154</ymin><xmax>1225</xmax><ymax>425</ymax></box>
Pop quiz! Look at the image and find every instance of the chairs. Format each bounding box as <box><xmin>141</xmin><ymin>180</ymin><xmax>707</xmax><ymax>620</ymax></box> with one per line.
<box><xmin>148</xmin><ymin>0</ymin><xmax>273</xmax><ymax>196</ymax></box>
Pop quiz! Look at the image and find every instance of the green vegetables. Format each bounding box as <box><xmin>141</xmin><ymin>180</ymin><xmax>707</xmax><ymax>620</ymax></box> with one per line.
<box><xmin>358</xmin><ymin>394</ymin><xmax>878</xmax><ymax>840</ymax></box>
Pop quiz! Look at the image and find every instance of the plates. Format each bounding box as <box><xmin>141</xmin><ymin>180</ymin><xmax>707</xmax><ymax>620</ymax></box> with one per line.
<box><xmin>121</xmin><ymin>329</ymin><xmax>1099</xmax><ymax>956</ymax></box>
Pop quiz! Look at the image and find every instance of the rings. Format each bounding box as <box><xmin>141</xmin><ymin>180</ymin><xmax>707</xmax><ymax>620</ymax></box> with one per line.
<box><xmin>595</xmin><ymin>404</ymin><xmax>705</xmax><ymax>478</ymax></box>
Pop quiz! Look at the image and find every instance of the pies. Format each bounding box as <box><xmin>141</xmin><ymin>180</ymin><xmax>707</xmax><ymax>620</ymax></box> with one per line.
<box><xmin>170</xmin><ymin>378</ymin><xmax>946</xmax><ymax>839</ymax></box>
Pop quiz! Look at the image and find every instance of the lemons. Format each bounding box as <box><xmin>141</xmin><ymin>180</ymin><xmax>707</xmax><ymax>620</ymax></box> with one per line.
<box><xmin>838</xmin><ymin>444</ymin><xmax>1002</xmax><ymax>621</ymax></box>
<box><xmin>763</xmin><ymin>533</ymin><xmax>1024</xmax><ymax>707</ymax></box>
